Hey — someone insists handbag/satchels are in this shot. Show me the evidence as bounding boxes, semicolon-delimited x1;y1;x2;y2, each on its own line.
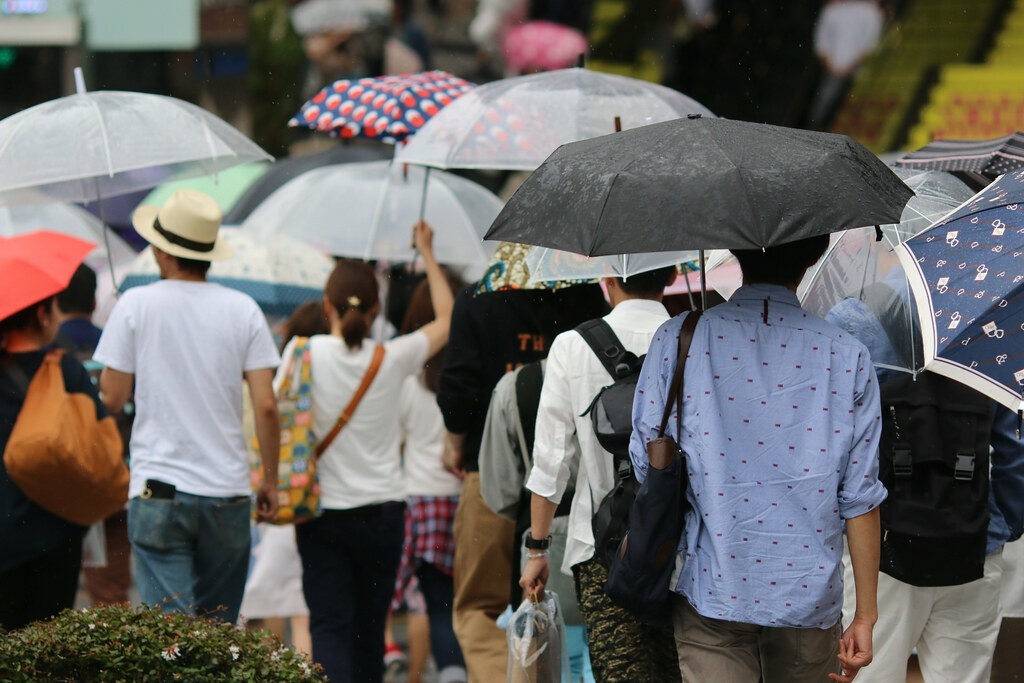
505;588;571;683
3;348;129;525
251;336;322;526
608;309;703;616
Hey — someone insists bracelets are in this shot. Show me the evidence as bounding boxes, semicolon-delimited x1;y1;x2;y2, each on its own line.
526;552;549;558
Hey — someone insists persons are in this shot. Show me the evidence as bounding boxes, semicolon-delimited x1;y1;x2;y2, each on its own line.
518;267;678;683
298;0;880;140
627;235;889;683
275;219;455;683
0;188;1024;683
93;188;283;626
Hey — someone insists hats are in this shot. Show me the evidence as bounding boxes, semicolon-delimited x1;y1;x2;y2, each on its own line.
133;189;233;261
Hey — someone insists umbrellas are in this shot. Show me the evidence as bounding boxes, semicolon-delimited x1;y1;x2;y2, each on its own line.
479;114;917;259
0;53;1024;416
0;67;276;204
243;159;511;267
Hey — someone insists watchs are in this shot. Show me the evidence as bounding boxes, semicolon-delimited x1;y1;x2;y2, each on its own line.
524;530;553;550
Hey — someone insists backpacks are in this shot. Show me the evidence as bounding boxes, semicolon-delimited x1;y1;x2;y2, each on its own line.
575;316;650;566
844;283;994;587
